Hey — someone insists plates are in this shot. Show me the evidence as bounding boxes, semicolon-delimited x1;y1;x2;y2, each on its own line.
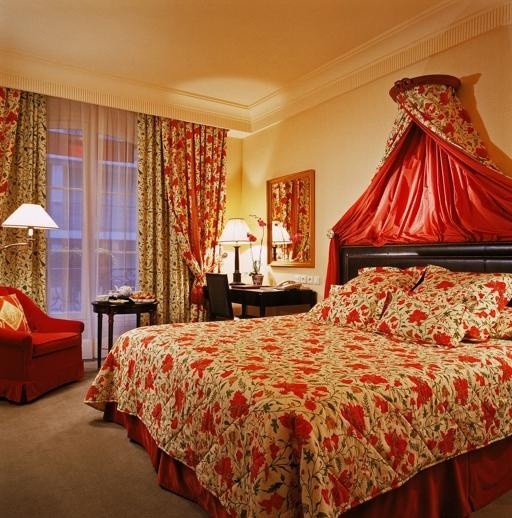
109;299;129;304
129;297;155;304
94;300;108;303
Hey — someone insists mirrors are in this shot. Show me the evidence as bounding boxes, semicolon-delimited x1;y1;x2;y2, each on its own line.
264;169;319;269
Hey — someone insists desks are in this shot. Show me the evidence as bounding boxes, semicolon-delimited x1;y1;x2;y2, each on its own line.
226;282;316;319
92;296;158;375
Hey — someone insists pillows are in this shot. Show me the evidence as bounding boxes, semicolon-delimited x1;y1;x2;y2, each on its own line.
412;265;511;339
311;283;389;332
373;287;489;348
305;264;426;323
488;306;511;341
0;288;33;332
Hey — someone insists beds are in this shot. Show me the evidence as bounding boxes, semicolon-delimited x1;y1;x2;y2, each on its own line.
82;232;512;516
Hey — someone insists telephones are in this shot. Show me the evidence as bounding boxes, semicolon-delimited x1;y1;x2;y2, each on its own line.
276;279;302;292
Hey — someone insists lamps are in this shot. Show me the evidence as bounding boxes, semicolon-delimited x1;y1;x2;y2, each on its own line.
0;201;60;257
271;220;292;260
215;218;254;285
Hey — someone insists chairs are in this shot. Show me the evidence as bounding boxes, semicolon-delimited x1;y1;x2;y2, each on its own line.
204;272;259;322
0;278;87;406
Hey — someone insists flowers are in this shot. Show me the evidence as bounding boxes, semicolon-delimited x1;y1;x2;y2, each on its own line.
246;214;266;270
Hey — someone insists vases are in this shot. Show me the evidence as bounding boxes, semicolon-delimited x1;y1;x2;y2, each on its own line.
252;273;265;288
